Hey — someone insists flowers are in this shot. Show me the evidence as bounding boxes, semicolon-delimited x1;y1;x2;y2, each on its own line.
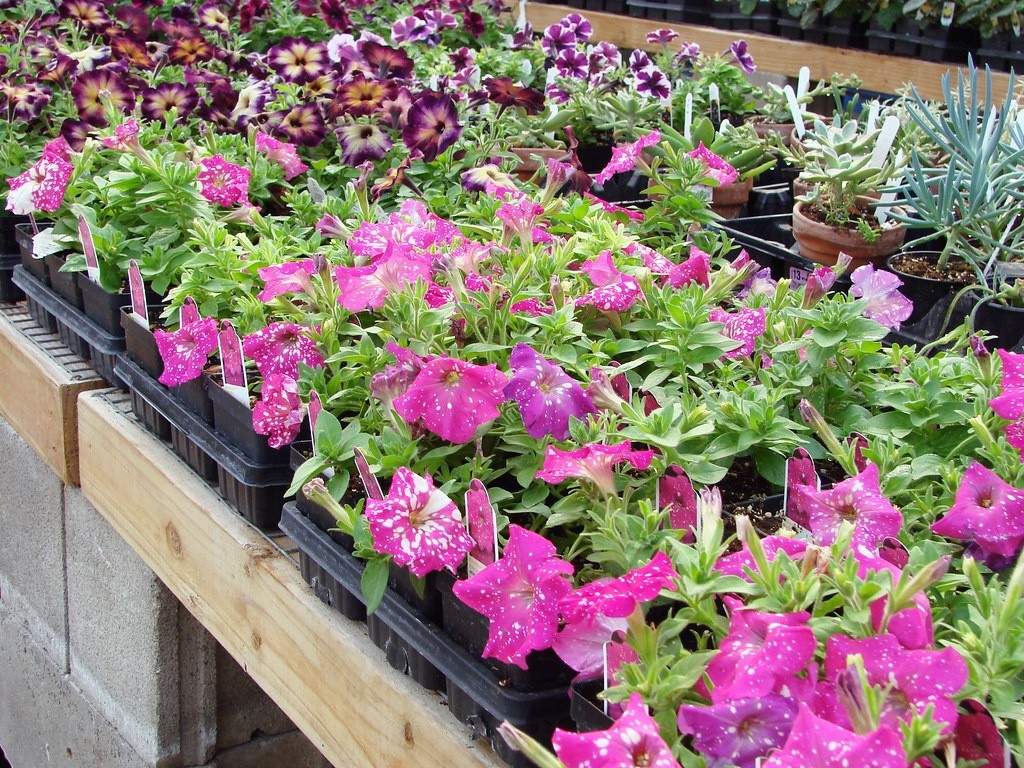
0;0;1024;768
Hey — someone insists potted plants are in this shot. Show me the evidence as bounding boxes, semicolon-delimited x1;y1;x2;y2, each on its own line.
484;51;1024;354
